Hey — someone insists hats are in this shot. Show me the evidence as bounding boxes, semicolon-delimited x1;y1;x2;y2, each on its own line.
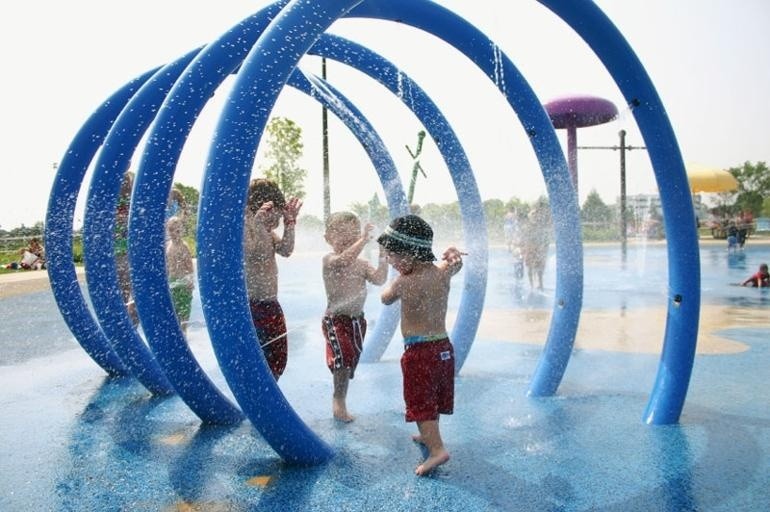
377;213;438;263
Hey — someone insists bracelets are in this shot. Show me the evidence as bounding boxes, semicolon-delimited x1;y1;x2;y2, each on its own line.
283;219;296;225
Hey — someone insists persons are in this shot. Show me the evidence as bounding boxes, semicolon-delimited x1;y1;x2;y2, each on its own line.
114;172;195;346
740;263;770;288
376;214;463;476
241;178;302;384
504;203;551;292
17;238;45;270
708;206;754;250
321;211;389;423
